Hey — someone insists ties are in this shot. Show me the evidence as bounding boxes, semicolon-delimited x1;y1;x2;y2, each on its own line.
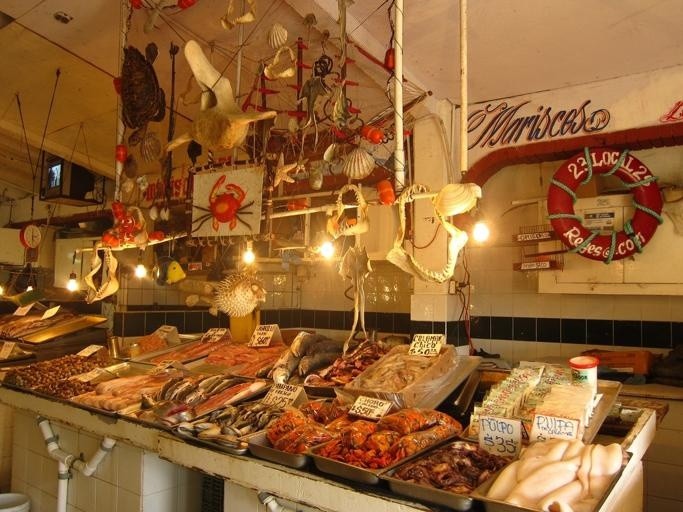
46;158;94;196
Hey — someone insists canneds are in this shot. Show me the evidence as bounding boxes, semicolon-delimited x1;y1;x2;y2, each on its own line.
547;148;663;260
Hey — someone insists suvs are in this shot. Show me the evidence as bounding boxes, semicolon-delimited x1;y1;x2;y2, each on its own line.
0;493;32;512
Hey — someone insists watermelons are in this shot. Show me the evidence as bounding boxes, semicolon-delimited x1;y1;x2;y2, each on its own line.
0;222;44;307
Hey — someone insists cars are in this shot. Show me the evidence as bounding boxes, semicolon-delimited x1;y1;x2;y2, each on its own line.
568;355;599;398
106;335;120;358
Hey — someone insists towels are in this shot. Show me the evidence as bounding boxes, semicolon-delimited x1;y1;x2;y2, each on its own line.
0;492;32;512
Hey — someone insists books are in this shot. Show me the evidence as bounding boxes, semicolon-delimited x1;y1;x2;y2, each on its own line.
342;342;458;413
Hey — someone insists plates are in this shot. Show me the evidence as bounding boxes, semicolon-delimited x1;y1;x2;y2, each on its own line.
536;191;683;296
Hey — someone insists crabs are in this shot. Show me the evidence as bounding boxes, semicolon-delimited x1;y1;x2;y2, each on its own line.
342;148;376;180
267;22;287;49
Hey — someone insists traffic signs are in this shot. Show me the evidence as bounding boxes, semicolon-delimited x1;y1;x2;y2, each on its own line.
449;280;457;295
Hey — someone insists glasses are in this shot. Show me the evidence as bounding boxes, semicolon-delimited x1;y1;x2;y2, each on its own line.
67;250;79;292
243;240;256;263
135;247;146;278
